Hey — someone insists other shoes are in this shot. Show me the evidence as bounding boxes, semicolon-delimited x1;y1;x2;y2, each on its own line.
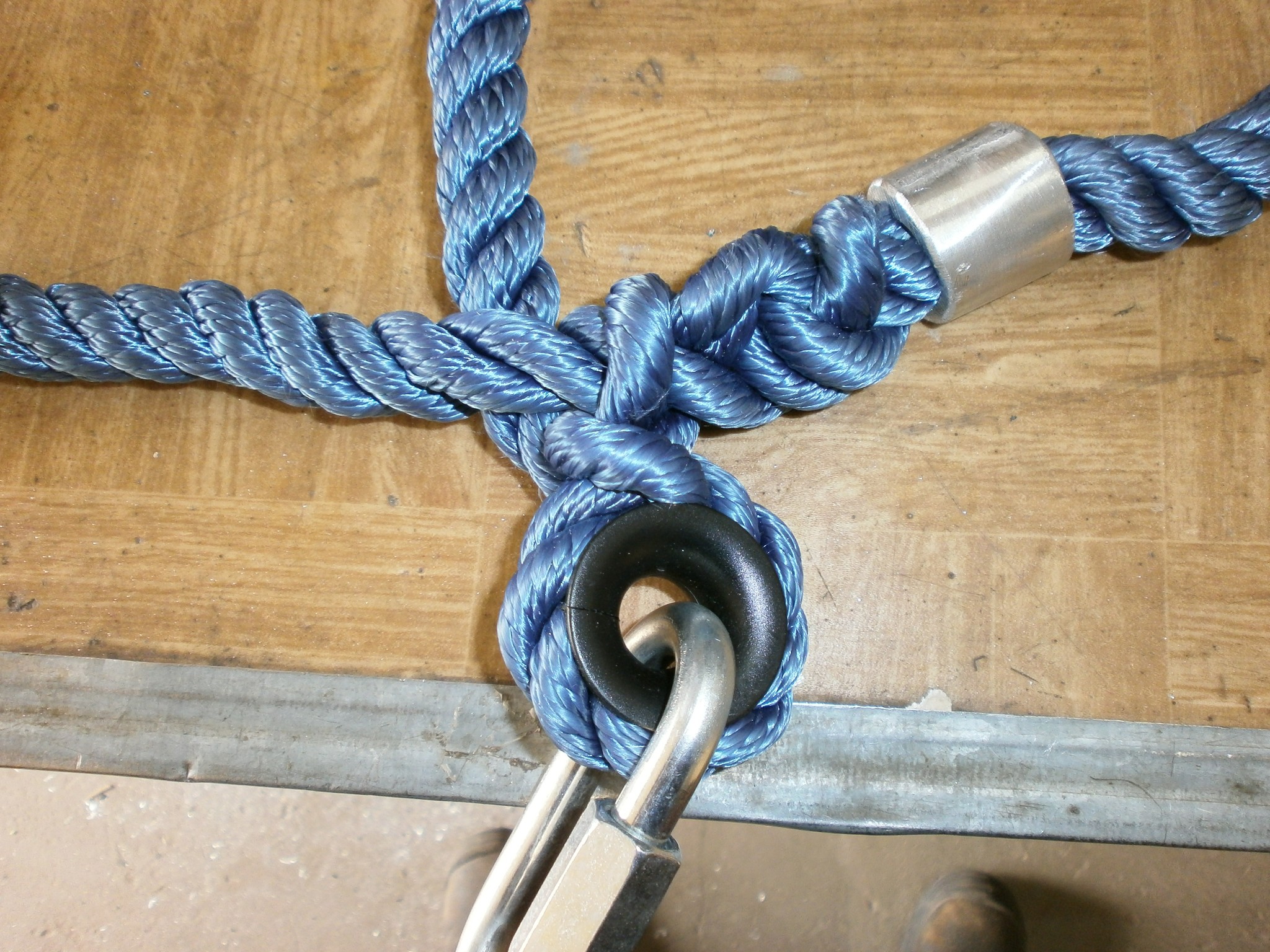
903;868;1025;952
440;827;512;952
